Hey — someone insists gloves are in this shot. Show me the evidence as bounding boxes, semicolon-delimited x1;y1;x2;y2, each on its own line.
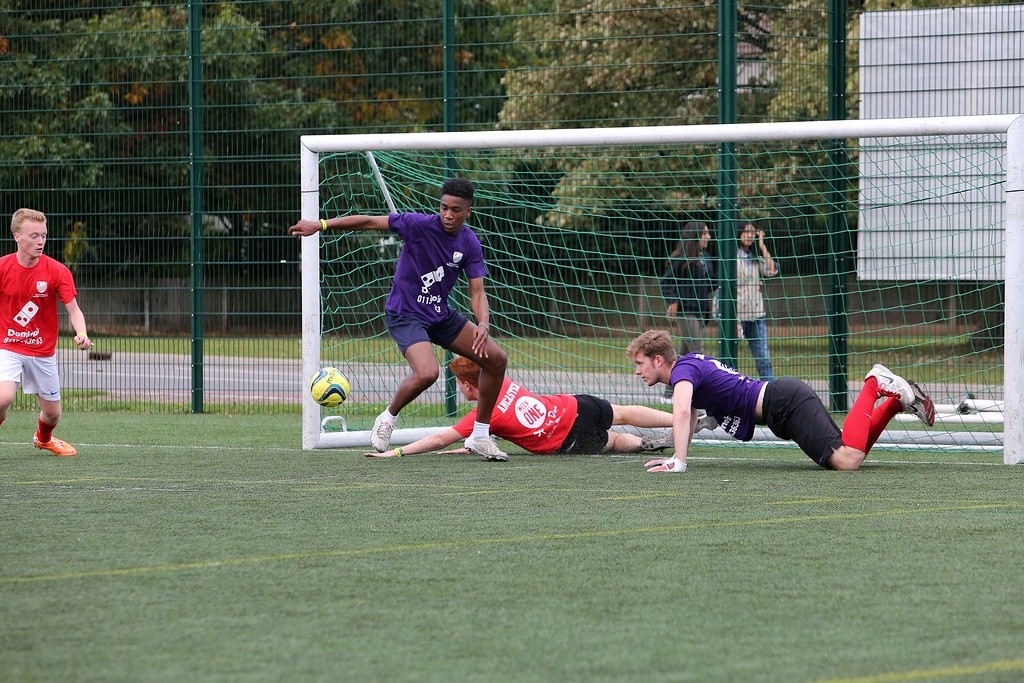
644;453;687;473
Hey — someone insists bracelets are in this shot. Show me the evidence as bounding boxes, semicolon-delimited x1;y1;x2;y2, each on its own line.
320;219;327;231
479;323;489;331
77;333;87;336
394;448;401;457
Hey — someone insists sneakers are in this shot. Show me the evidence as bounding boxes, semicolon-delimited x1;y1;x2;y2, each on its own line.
897;380;935;426
370;412;397;452
864;363;915;405
464;434;509;461
33;432;77;456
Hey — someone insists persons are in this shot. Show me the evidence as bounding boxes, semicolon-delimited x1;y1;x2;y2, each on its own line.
288;180;510;462
715;222;778;381
365;356;718;457
627;330;934;473
661;221;719;403
0;208;91;455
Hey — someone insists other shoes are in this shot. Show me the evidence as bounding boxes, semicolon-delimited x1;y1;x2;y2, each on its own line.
661;397;673;405
640;429;674;451
694;411;717;433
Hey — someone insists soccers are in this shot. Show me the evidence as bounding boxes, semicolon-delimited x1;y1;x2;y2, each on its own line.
310;366;349;407
